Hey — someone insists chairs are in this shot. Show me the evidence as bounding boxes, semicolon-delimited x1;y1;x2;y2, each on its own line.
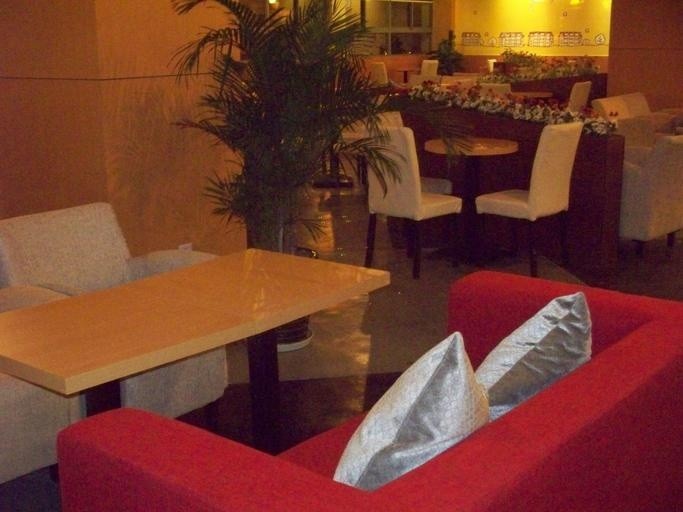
472;119;584;278
364;122;464;281
567;79;683;256
1;203;227;482
0;280;83;486
359;111;451;249
366;58;553;104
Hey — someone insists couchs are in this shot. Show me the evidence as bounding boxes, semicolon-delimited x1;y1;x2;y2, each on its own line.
58;269;683;512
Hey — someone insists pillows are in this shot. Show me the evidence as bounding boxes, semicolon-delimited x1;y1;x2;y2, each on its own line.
333;332;494;493
473;290;592;422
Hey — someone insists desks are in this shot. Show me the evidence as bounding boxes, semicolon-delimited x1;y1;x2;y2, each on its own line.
419;130;519;164
0;250;390;459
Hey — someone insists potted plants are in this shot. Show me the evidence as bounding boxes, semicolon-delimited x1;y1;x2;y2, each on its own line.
158;0;470;352
